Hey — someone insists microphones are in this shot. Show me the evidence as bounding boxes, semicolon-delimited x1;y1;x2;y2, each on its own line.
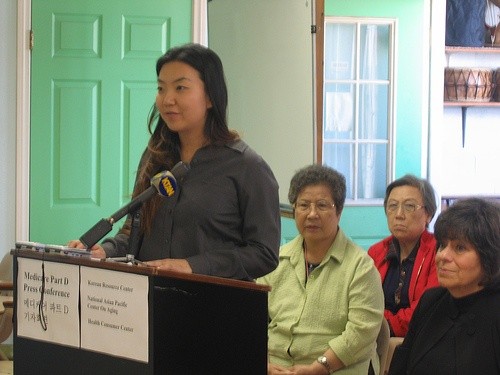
79;159;192;249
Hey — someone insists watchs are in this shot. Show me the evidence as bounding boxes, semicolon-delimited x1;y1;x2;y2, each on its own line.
317;356;333;375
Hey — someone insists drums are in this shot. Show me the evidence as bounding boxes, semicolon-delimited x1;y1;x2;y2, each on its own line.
443;66;496;102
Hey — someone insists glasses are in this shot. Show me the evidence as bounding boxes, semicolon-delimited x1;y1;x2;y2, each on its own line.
294;198;335;212
393;263;409;305
385;199;426;212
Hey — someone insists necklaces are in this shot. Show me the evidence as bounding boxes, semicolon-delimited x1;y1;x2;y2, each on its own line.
304;244;309;276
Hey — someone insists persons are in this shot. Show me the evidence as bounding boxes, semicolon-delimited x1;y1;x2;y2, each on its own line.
256;164;385;375
390;198;500;375
368;175;440;337
68;43;281;280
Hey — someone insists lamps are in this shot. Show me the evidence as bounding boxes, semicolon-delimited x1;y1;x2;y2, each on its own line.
442;67;500;148
